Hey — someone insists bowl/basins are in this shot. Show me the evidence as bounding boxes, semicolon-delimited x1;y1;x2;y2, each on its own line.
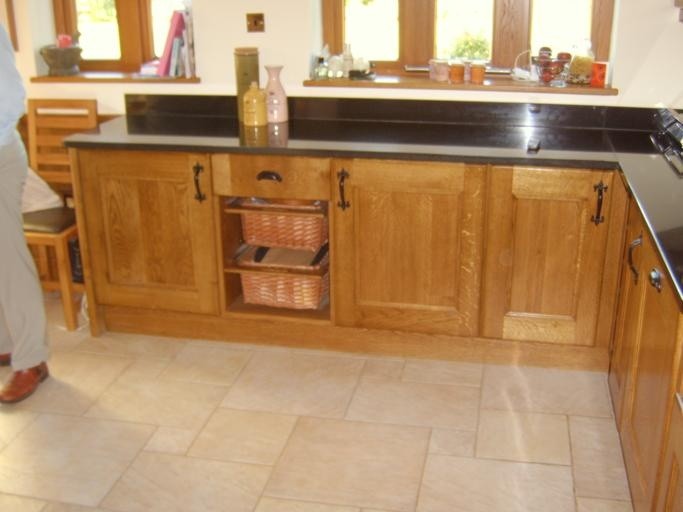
530;56;571;87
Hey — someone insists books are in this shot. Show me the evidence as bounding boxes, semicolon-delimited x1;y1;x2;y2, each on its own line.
156;9;195;80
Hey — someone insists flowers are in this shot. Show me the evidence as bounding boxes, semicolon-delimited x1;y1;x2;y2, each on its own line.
55;30;85;48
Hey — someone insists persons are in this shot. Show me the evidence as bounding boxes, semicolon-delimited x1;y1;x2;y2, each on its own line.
0;25;51;405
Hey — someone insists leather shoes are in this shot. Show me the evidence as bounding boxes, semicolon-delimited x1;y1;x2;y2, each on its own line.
1;352;48;403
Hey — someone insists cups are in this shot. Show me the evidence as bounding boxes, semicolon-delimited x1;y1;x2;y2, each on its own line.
470;64;485;83
450;64;464;83
428;60;450;82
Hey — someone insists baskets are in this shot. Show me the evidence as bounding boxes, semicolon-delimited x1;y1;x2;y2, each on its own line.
236;254;329;310
238;200;329;252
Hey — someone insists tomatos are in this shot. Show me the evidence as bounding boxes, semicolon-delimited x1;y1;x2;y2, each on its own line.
539;47;570;83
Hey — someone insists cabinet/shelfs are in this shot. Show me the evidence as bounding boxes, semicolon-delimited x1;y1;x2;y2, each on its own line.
330;157;486;339
600;197;682;510
72;147;220;318
208;152;336;322
478;165;616;351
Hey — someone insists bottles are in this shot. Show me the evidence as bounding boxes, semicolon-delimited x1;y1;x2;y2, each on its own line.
344;43;354;79
234;47;259;123
312;57;328;80
265;64;288;123
243;81;268;127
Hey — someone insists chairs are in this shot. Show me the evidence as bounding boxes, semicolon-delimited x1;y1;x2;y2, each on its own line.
8;93;103;333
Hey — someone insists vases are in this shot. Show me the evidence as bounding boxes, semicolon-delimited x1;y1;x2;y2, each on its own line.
39;45;81;78
